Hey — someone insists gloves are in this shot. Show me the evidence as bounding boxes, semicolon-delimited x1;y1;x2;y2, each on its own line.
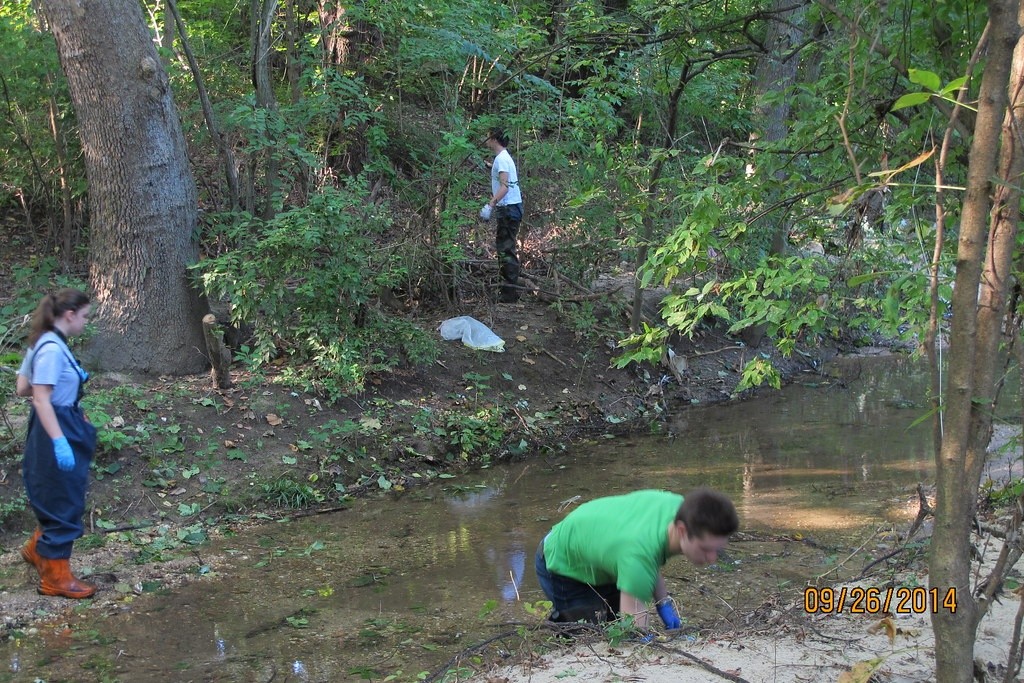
52;436;75;473
655;600;680;630
477;203;494;223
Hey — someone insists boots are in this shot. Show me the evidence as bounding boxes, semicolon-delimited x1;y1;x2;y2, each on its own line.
20;524;43;566
37;555;98;598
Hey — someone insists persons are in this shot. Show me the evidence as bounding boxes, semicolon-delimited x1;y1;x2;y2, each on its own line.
535;489;740;645
479;129;524;303
15;287;96;598
